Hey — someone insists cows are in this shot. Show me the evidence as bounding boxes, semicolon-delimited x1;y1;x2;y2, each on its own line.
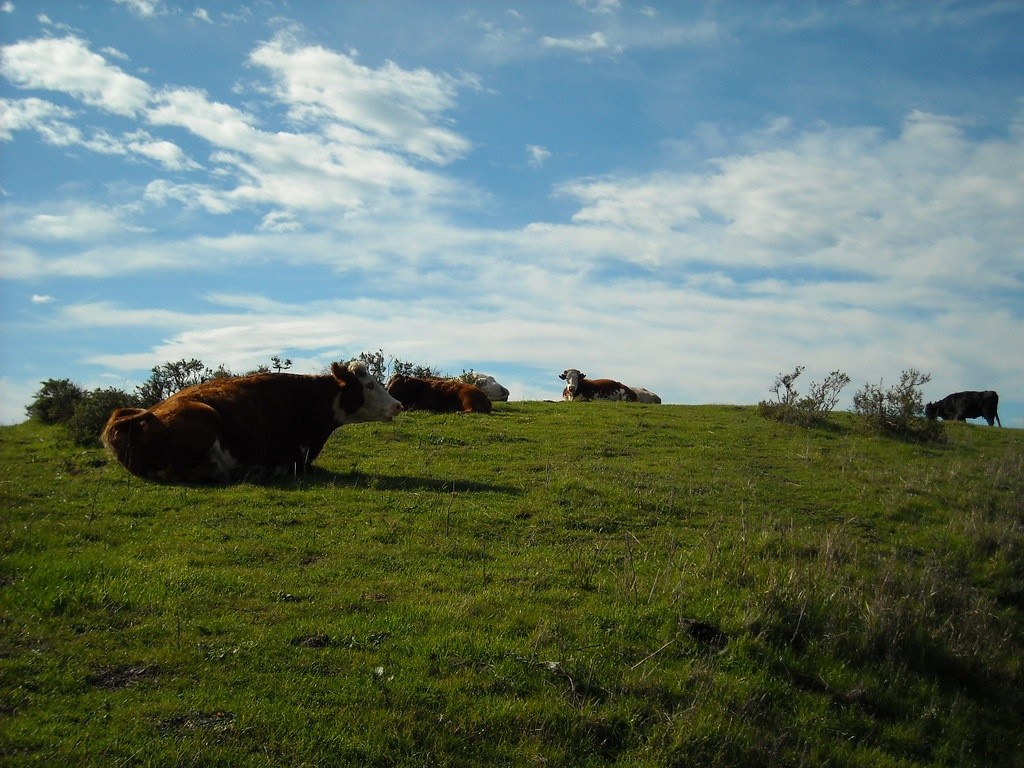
387;373;508;422
558;368;661;403
99;360;405;492
925;390;1001;427
